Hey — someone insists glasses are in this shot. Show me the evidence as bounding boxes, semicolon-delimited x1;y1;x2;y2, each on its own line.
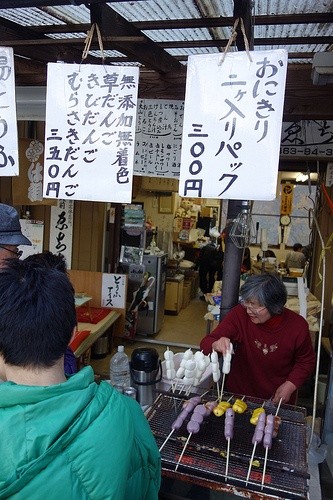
240;298;267;314
0;246;23;259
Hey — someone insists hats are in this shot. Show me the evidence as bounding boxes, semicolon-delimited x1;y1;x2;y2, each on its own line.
0;203;32;246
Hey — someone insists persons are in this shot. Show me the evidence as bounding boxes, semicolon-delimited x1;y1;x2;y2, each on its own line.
285;243;314;274
0;257;164;500
243;248;252;272
200;271;317;403
0;203;79;378
193;241;224;301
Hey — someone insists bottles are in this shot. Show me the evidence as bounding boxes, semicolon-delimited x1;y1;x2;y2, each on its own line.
110;345;131;393
129;348;163;406
124;387;136;400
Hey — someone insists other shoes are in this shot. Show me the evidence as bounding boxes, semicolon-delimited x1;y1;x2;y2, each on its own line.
200;295;206;301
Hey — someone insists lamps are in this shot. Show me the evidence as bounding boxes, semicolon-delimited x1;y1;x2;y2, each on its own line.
310;51;333;86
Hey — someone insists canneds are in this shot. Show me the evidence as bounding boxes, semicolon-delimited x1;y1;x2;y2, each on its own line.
122;387;137;400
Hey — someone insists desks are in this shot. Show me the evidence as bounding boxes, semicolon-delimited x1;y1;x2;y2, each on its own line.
67;305;126;359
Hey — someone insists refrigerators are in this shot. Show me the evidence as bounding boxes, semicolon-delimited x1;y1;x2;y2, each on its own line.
136;253;167;336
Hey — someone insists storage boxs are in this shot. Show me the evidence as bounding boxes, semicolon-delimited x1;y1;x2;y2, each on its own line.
201;207;213;217
164;271;200;316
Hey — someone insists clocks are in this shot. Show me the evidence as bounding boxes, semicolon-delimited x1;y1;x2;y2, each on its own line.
280;214;291;226
